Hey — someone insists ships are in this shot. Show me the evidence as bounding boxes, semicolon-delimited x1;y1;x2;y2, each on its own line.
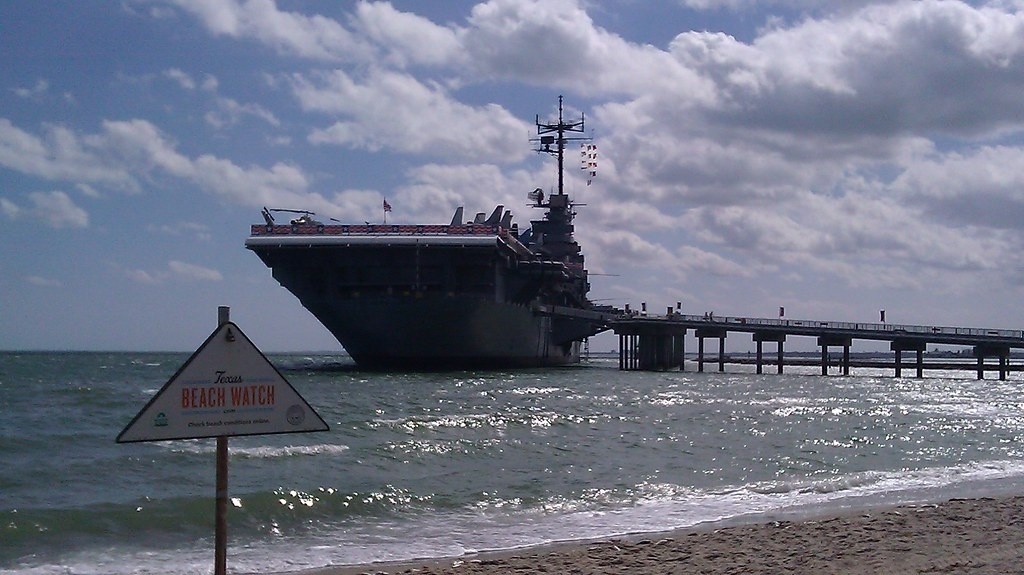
243;94;624;365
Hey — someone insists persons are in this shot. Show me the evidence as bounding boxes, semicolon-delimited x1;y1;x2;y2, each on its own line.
705;311;713;323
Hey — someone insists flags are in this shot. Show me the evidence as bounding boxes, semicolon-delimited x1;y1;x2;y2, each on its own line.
384;200;392;212
581;142;599;186
